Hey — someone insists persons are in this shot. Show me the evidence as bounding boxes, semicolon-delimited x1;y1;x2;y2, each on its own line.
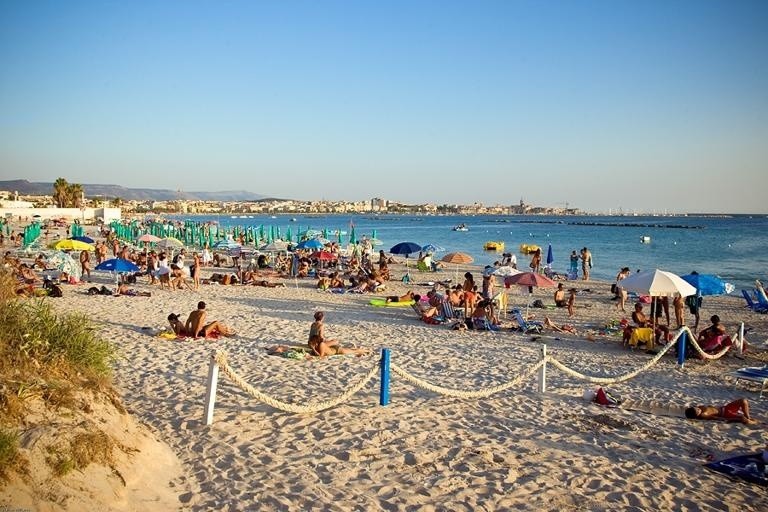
109;230;201;296
555;247;592;319
168;301;236;340
308;311;373;358
615;267;630;313
201;234;390;294
619;297;750;352
684;398;766;425
385;253;517;326
532;248;542;273
2;215;119;297
685;270;703;331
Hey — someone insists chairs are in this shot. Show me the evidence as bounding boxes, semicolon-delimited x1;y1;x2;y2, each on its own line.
728;364;768;396
410;301;456;318
490;292;503;319
741;280;768;315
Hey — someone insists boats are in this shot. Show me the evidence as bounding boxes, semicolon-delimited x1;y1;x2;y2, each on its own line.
322;229;349;235
453;227;470;232
519;243;542;255
483;239;505;251
